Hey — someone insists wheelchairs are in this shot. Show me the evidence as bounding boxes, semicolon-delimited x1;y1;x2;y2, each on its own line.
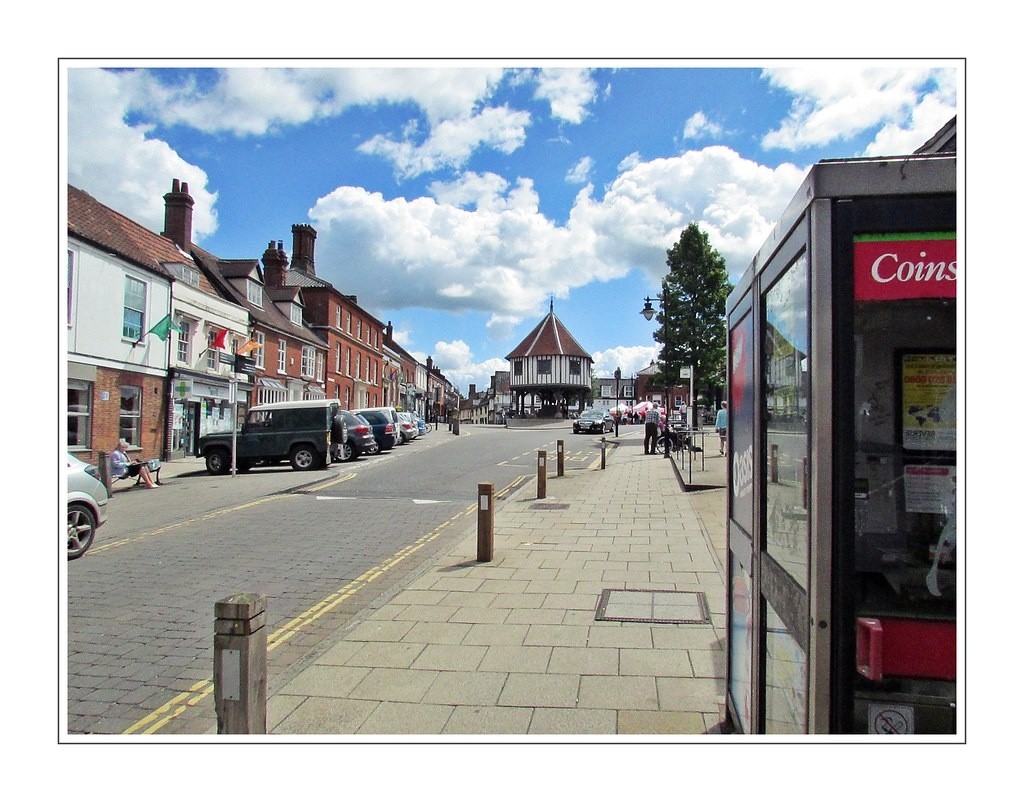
656;422;688;454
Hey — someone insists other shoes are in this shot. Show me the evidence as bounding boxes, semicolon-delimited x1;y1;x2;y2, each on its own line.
650;452;657;455
145;483;160;488
720;449;724;453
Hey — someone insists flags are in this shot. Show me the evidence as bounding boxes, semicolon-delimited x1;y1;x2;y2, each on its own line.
390;369;404;382
149;313;183;341
211;328;226;350
235;338;263;356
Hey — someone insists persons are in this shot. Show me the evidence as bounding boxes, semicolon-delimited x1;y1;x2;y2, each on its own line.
659;415;679;452
109;441;160;489
714;400;727;457
607;406;648;425
644;402;661;455
679;401;688;423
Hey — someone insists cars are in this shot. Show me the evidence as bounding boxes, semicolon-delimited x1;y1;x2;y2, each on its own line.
337;409;376;463
397;412;427;446
354;408;398;456
572;407;616;434
67;451;110;554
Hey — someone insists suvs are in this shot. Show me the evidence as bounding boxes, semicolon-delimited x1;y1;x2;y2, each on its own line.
196;399;347;476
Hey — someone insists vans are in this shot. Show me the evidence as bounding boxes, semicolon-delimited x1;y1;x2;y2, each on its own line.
370;406;400;446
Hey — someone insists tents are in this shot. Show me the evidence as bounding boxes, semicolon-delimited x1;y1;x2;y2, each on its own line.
633;401;664;412
610;403;632;414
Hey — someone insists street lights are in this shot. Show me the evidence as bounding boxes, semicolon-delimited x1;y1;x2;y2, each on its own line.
638;289;671;458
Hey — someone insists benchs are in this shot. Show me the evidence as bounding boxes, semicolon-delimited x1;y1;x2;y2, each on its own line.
111;466;162;485
